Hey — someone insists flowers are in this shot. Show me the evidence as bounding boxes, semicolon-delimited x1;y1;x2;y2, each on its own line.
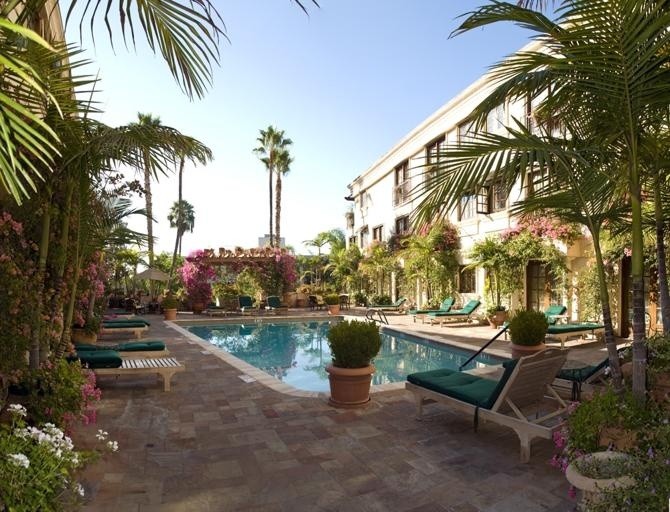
545;380;670;512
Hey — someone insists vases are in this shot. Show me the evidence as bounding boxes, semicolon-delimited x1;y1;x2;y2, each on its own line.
565;451;635;512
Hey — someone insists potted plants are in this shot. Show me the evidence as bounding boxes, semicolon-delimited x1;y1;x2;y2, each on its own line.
324;320;388;409
324;295;341;315
161;293;177;320
509;308;549;366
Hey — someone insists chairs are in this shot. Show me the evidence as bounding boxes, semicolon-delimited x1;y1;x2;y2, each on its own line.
504;305;605;347
406;346;629;466
206;296;288;317
65;308;185;391
366;298;480;327
309;294;350;312
